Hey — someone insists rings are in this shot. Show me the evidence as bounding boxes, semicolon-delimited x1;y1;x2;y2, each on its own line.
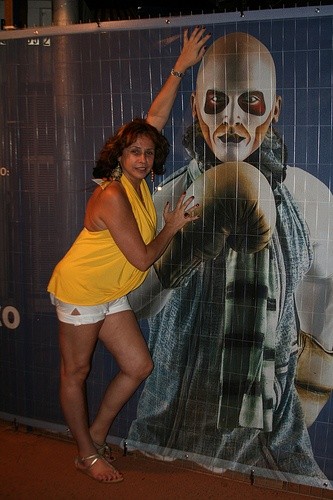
182;212;189;218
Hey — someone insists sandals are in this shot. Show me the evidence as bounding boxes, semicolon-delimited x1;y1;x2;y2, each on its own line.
73;453;124;483
92;440;116;462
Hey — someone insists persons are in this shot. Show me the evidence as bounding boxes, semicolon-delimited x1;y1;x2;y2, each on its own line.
126;31;333;483
45;26;212;484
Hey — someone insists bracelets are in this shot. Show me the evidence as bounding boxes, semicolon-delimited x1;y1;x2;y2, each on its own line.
170;68;183;78
